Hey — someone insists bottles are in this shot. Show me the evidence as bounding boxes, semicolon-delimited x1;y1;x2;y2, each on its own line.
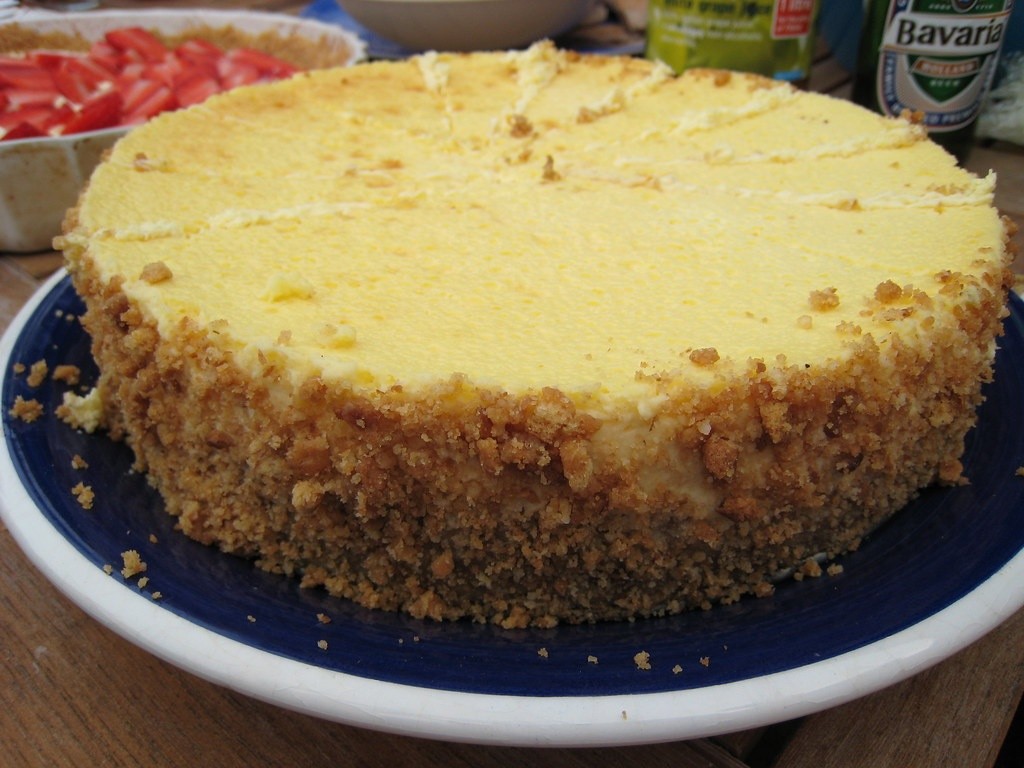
642;0;821;96
853;0;1016;171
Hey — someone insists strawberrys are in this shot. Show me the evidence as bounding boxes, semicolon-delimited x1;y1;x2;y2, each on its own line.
0;23;301;143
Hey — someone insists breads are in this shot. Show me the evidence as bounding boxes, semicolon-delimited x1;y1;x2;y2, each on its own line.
54;36;1016;635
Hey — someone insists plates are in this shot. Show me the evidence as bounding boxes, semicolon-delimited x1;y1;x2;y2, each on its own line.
0;216;1023;755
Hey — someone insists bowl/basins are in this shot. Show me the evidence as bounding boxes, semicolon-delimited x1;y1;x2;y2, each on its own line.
0;9;364;258
330;0;595;51
